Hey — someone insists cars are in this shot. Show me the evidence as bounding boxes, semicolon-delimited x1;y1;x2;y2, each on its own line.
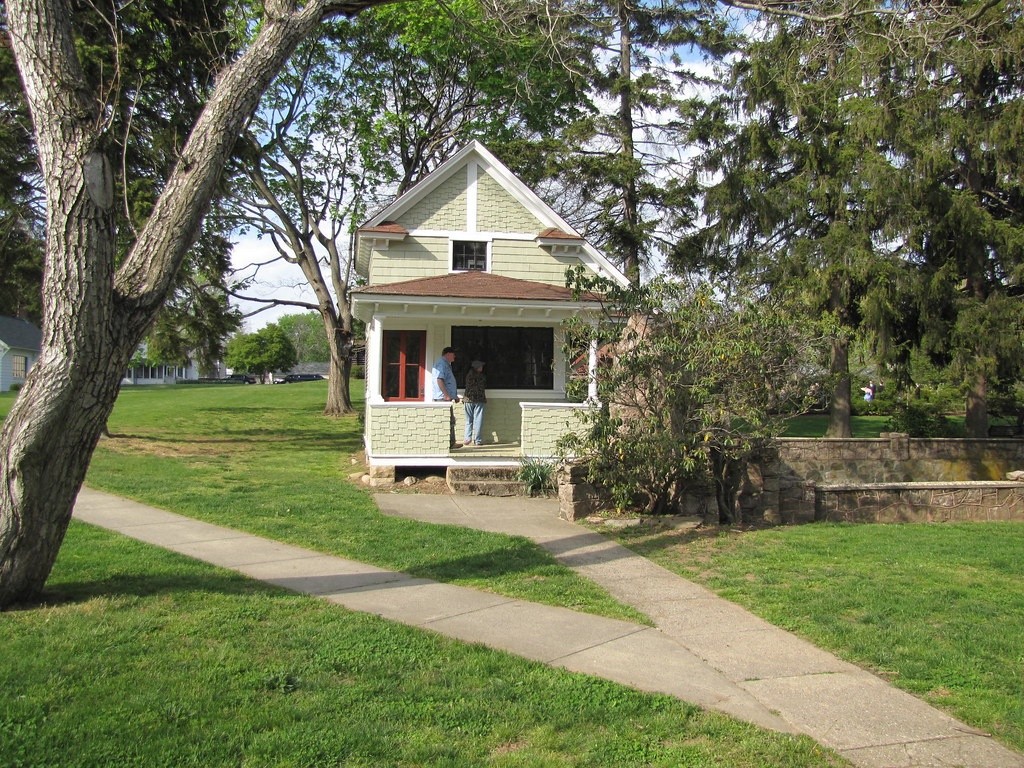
272;378;286;385
230;374;256;384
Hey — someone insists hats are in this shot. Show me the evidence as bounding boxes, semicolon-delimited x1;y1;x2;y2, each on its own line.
442;347;456;356
471;360;485;369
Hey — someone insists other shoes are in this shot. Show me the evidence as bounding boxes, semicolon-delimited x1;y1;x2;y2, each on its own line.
464;439;471;445
475;443;483;445
451;443;463;449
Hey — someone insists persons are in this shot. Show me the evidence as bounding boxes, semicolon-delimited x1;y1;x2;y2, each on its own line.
915;383;921;400
861;379;884;401
431;347;464;449
458;334;494;389
463;360;488;446
